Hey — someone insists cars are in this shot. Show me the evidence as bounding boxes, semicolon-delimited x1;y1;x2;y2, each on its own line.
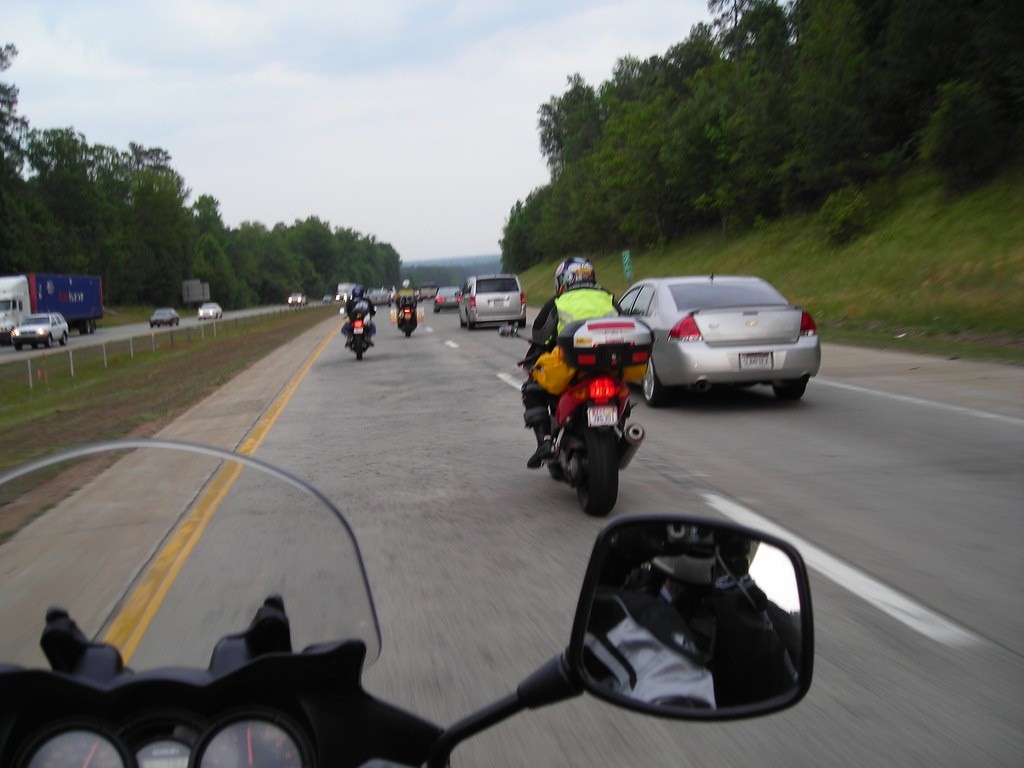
616;274;821;408
414;283;464;313
322;281;357;303
369;288;392;307
148;307;180;328
198;302;223;319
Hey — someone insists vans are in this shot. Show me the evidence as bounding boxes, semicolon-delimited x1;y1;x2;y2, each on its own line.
455;273;526;332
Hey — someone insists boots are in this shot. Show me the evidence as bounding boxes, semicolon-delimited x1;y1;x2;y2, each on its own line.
527;419;555;469
366;334;374;346
345;332;353;346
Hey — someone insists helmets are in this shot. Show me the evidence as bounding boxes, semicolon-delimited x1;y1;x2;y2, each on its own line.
402;279;411;289
554;257;595;295
352;286;364;297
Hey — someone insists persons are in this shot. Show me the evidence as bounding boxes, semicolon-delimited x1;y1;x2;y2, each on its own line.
521;255;628;470
393;279;417;328
341;286;376;347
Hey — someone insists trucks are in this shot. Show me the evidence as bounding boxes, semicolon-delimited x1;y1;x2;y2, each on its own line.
0;272;104;345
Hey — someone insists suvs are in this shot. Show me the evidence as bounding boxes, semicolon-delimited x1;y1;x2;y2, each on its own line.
288;291;312;308
11;313;69;350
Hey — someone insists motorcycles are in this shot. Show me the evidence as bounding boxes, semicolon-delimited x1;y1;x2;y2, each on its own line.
396;296;418;338
347;306;377;360
528;315;656;517
